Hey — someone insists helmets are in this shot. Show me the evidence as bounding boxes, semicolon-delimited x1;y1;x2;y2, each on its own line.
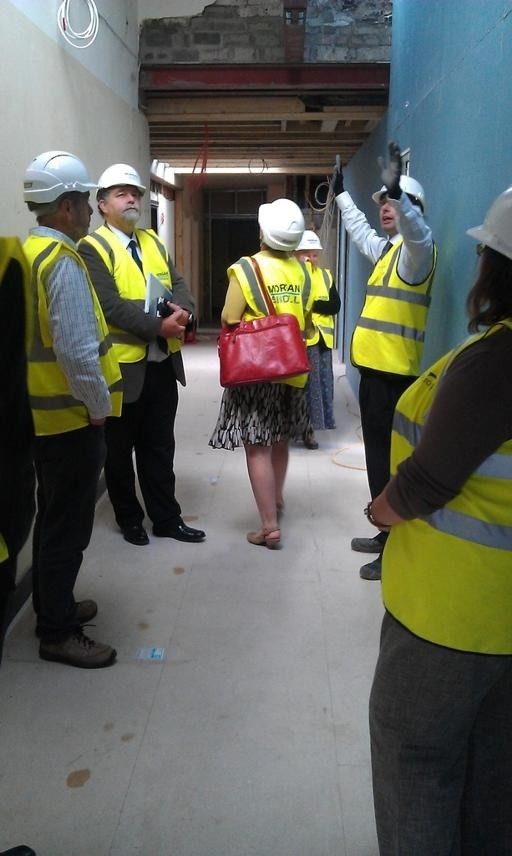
466;187;512;259
258;199;305;252
21;150;104;214
371;175;425;212
95;163;147;203
296;230;323;251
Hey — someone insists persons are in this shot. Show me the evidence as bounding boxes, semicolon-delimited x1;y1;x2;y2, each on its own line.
76;164;208;544
372;185;509;849
212;199;306;549
285;229;340;449
327;142;434;580
19;151;126;670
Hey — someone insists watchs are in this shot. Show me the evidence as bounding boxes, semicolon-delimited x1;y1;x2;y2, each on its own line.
364;501;392;527
188;309;195;328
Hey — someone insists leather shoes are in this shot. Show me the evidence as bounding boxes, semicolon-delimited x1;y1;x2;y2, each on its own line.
123;525;149;545
303;431;318;450
153;521;207;542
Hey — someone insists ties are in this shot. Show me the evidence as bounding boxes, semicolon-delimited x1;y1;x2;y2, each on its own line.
129;241;143;273
380;241;393;256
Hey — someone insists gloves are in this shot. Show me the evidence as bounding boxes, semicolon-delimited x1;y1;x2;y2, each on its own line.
378;141;403;198
326;154;344;196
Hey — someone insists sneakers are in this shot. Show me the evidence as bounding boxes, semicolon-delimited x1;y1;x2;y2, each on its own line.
38;633;117;670
74;599;98;622
352;535;383;552
360;555;384;580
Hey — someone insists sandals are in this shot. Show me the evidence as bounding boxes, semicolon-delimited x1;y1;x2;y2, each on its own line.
276;503;284;520
245;527;283;548
